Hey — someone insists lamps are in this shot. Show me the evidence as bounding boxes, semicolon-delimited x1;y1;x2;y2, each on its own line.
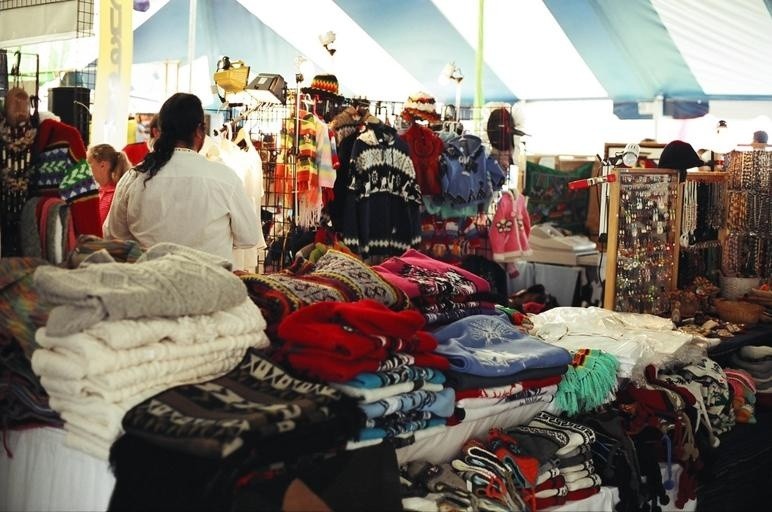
242;73;287;105
438;59;464;120
622;143;640;168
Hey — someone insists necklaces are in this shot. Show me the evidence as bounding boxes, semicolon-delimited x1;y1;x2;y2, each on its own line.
679;150;772;280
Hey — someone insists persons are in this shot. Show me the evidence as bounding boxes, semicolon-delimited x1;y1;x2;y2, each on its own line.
121;112;161;167
86;143;129;226
751;131;768;144
106;92;260;271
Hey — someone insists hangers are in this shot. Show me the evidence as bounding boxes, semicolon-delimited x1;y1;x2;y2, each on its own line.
225;113;253;148
305;93;313;113
434;121;475;146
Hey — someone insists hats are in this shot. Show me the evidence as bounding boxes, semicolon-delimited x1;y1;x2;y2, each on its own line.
401;410;603;512
563;362;757;512
401;93;440;123
301;75;344;103
658;141;704;169
